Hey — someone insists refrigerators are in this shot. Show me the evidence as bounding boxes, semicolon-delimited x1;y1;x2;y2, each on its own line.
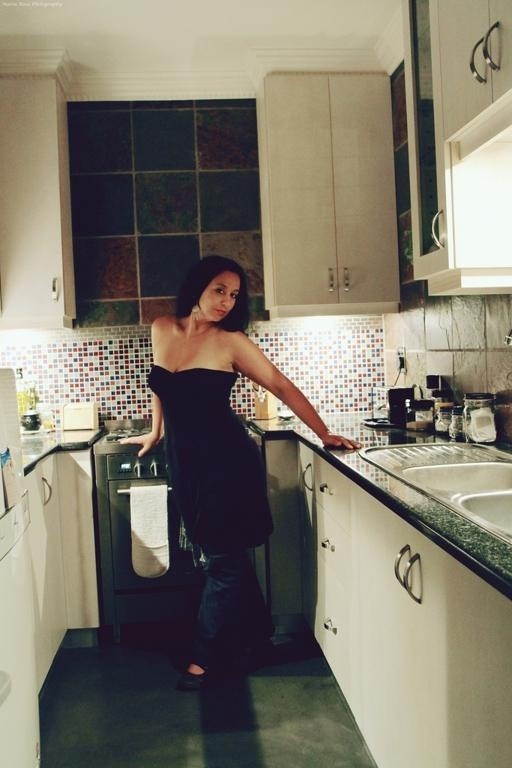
0;364;42;767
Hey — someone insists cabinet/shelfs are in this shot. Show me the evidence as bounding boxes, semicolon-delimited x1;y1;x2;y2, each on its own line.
402;1;512;296
251;67;402;317
0;49;79;336
253;429;349;705
436;1;512;162
25;445;100;701
350;486;511;767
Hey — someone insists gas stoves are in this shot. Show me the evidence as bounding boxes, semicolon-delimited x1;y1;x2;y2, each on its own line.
93;426;264;480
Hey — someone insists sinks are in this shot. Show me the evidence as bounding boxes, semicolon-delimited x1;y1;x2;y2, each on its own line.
398;461;512;496
451;491;512;546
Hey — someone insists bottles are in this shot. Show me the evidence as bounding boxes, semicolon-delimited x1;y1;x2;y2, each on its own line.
426;374;439;402
462;391;512;443
431;388;442;422
406;399;433;431
449;406;465;439
436;404;450;432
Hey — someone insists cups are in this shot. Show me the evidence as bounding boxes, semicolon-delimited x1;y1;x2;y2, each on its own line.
20;413;40;432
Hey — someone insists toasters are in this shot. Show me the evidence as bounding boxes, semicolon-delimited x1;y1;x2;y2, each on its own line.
364;384;415;429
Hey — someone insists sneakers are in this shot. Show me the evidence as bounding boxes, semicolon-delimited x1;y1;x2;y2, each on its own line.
177;663;212;690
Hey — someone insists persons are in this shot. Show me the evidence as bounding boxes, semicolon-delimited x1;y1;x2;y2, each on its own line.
117;254;366;694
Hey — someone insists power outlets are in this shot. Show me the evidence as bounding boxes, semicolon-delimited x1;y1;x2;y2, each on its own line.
396;345;408;374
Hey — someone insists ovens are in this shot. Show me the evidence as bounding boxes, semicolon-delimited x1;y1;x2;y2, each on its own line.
93;481;267;647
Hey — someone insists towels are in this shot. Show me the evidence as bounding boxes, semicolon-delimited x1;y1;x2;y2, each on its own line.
128;485;170;579
